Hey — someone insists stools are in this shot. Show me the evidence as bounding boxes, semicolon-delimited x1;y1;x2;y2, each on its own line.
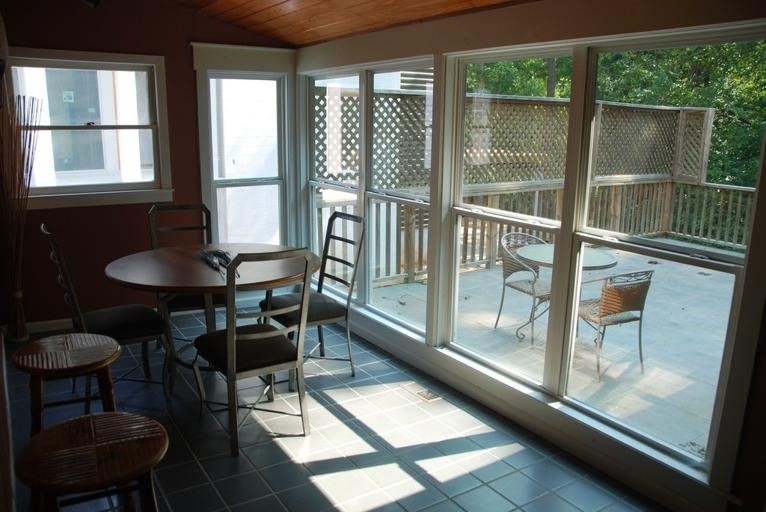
9;333;173;510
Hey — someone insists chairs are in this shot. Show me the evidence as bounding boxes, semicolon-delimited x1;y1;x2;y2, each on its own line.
258;210;370;392
193;248;314;459
148;203;227;349
492;232;655;380
42;223;172;415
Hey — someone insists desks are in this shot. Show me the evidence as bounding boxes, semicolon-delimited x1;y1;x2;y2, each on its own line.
104;243;321;422
